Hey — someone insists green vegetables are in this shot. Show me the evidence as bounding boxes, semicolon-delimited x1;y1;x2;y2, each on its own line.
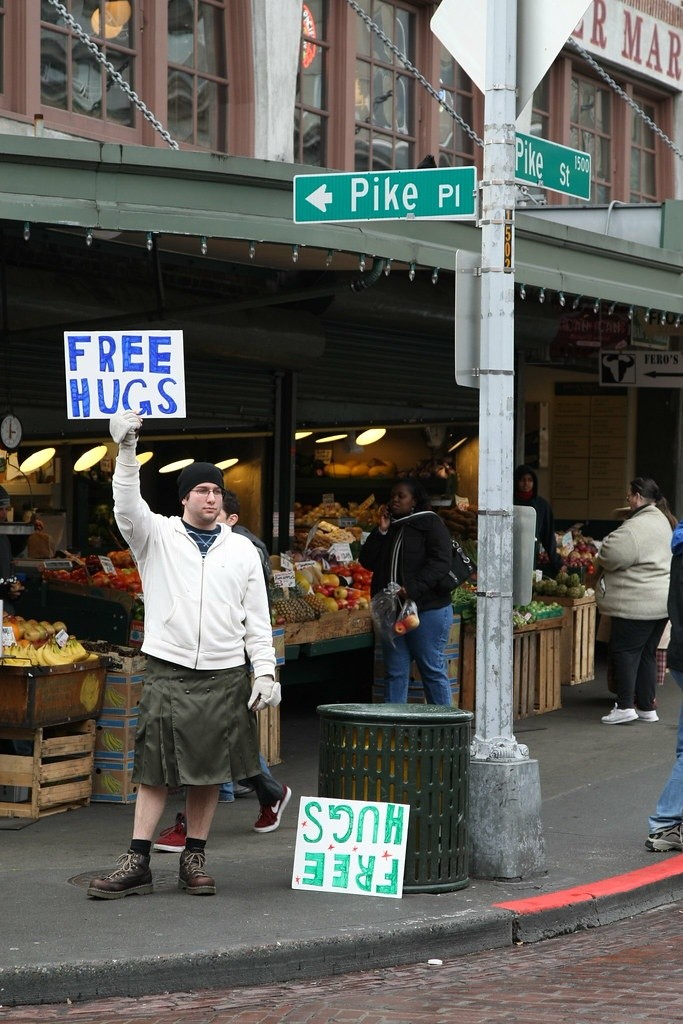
514;600;561;629
450;532;478;604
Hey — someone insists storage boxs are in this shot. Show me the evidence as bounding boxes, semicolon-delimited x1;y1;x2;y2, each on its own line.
318;516;356;527
250;627;285;673
283;607;373;646
75;639;146;804
0;718;97;820
370;613;461;708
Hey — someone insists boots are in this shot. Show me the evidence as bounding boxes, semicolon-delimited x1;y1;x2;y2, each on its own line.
87;849;153;899
178;847;217;894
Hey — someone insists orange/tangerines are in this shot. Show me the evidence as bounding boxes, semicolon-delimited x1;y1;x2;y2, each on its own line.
135;605;144;621
4;623;20;641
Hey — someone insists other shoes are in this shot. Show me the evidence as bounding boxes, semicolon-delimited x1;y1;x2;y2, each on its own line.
233;783;255;795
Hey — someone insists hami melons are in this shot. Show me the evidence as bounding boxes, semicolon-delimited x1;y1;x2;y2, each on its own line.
329;458;393;476
269;553;322;574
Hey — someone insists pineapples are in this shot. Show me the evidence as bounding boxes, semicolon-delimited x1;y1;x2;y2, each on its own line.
269;581;329;623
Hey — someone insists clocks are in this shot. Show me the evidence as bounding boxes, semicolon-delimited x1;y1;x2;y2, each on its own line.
0;413;23;451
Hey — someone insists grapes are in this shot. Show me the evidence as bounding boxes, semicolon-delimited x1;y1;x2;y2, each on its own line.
81;641;148;659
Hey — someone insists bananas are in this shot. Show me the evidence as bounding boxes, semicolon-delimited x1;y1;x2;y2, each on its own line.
2;635;98;666
102;686;127;794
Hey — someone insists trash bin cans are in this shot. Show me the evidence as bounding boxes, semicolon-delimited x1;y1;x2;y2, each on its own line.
317;704;475;894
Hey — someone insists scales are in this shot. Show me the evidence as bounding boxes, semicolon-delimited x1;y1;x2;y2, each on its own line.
15;557;73;571
0;413;36;534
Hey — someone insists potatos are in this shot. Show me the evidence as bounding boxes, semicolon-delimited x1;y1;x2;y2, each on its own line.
437;509;477;531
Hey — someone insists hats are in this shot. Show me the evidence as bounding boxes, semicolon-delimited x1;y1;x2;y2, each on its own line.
177;462;226;502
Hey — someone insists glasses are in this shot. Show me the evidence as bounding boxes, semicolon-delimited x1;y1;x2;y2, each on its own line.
190;488;225;496
626;493;635;501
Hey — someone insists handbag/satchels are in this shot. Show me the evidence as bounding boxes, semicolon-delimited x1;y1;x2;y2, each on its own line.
424;529;474;598
368;582;419;648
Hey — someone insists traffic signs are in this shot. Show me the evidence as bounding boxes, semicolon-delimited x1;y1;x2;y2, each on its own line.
294;165;480;224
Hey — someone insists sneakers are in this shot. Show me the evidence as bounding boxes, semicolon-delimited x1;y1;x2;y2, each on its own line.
253;784;292;833
645;823;683;852
153;812;187;852
635;707;659;722
601;702;639;724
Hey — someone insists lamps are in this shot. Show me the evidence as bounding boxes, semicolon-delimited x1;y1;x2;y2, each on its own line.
314;428;387;446
71;443;108;472
156;445;194;473
206;440;239;470
18;446;56;472
446;431;469;453
116;442;153;467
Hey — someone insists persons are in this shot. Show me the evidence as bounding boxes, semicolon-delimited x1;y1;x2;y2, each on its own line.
88;411;274;895
154;490;292;853
359;479;454;705
513;465;560;567
594;478;678;725
0;485;43;614
645;517;683;850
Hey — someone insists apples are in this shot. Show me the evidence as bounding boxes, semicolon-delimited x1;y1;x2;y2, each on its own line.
294;502;388;525
44;549;143;592
312;562;373;610
539;543;597;574
393;614;419;635
3;608;67;641
